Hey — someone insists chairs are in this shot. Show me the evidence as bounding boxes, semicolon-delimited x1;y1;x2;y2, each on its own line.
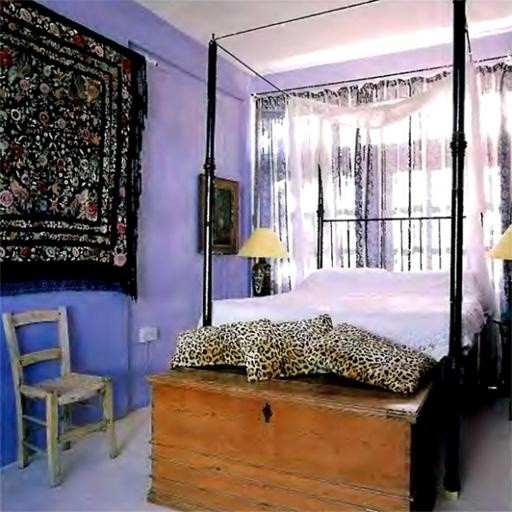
2;305;117;489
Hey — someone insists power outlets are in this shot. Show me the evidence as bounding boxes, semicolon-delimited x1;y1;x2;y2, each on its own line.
138;325;159;343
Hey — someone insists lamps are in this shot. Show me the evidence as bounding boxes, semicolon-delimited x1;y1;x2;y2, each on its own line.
487;222;512;319
237;226;291;297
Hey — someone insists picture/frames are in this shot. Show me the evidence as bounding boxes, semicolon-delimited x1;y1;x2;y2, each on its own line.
199;173;240;254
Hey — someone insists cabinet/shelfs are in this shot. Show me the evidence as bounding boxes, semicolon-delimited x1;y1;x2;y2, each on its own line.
142;363;444;512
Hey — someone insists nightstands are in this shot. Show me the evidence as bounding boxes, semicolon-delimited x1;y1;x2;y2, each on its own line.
484;313;512;422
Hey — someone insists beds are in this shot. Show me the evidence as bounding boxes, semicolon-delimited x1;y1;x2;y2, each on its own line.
201;0;495;501
